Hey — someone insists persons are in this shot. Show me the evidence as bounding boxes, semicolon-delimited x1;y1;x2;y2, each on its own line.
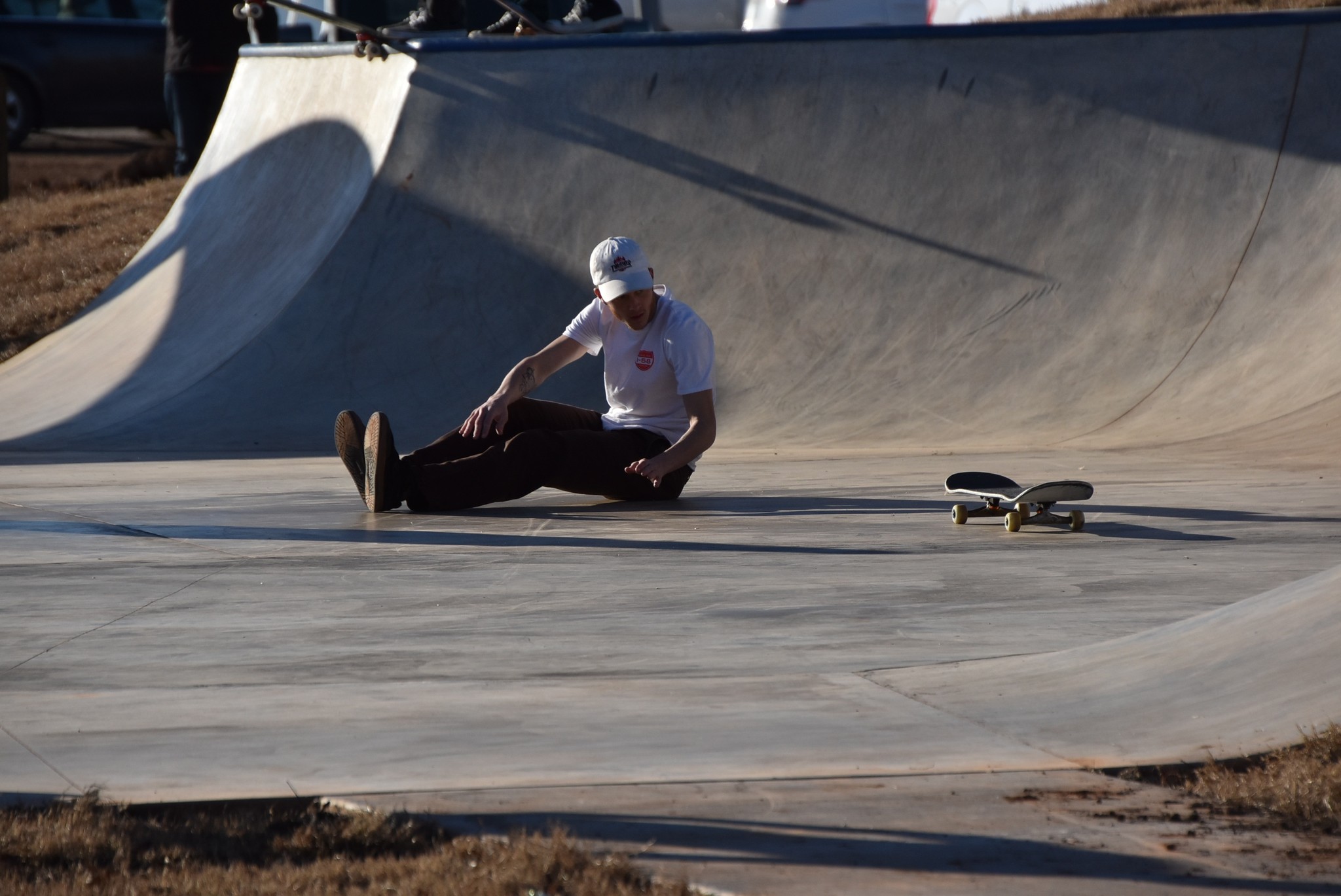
334;236;717;515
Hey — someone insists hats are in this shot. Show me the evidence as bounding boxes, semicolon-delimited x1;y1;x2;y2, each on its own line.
587;236;655;304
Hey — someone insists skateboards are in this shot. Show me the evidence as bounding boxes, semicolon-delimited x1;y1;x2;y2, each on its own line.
945;471;1094;532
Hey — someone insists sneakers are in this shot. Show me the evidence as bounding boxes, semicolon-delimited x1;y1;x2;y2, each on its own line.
334;409;367;507
465;9;519;38
362;411;404;514
377;6;469;41
544;0;623;34
